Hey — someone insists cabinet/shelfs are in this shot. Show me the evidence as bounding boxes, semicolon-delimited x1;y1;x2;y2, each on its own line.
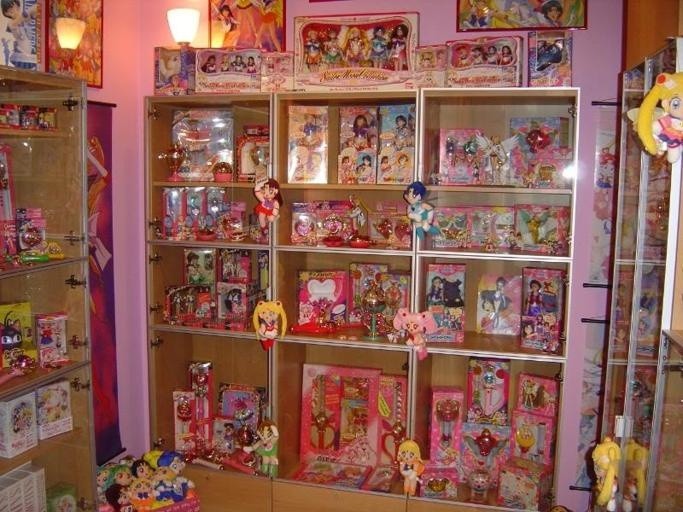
271;90;420;500
142;93;274;481
0;64;101;512
409;87;582;512
570;34;683;512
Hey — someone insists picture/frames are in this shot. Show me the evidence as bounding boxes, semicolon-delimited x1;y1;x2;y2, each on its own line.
207;0;287;53
44;0;104;89
454;0;589;33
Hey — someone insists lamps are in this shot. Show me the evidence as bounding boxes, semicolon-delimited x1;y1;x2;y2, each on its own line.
164;6;202;45
51;11;89;62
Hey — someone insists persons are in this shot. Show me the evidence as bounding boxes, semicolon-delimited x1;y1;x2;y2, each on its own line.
592;436;620;512
627;71;683;163
393;308;437;361
96;449;196;512
243;419;280;479
622;440;647;511
403;181;441;241
254;164;283;236
253;300;287;351
396;440;425;495
